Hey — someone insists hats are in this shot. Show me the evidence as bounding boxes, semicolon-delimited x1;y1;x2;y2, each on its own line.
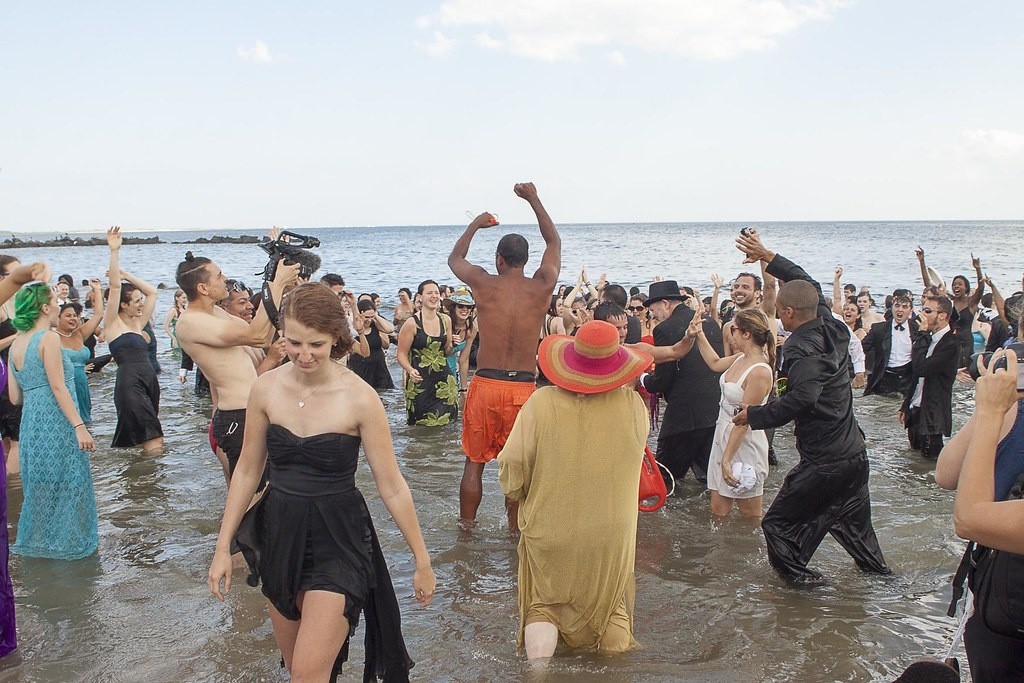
538;320;654;393
443;287;476;312
643;280;691;308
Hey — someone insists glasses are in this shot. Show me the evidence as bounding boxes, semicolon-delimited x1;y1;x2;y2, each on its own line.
921;307;942;314
572;306;588;314
229;281;244;295
628;305;647;311
455;304;472;309
335;291;345;298
730;325;743;336
910;297;914;302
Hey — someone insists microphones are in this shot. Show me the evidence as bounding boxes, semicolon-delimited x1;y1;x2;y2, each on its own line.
277;244;321;271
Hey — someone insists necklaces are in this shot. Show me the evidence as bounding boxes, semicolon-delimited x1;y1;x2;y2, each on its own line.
288;366;337;408
56;331;71;338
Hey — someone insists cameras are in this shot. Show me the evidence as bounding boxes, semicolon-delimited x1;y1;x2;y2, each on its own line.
968;340;1024;392
734;407;743;416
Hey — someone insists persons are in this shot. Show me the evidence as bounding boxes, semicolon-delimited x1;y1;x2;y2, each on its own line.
637;280;727;485
498;319;651;667
0;354;17;659
0;226;1024;513
205;283;436;683
935;348;1024;682
7;282;98;559
696;309;774;517
448;182;562;532
174;251;300;506
733;229;889;582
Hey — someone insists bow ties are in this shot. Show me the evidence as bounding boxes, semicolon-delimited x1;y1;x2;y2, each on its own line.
894;323;905;330
